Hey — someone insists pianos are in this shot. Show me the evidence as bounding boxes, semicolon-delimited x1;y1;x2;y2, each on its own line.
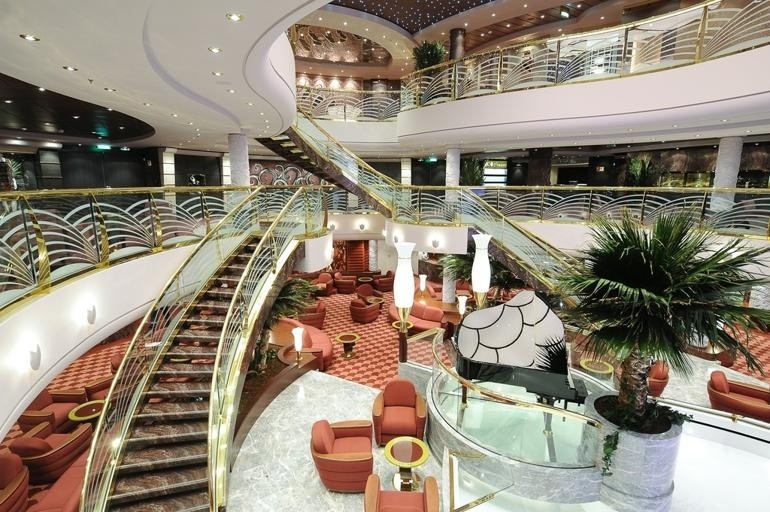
443;286;588;430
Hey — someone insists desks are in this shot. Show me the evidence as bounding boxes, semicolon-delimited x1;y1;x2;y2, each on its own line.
335;333;361;359
391;320;414;342
580;359;614;381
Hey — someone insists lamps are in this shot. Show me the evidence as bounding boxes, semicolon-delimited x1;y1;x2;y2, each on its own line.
471;232;492;311
393;241;415;364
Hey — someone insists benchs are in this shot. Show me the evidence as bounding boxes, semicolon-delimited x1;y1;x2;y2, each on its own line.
389;300;444;341
295;320;333;371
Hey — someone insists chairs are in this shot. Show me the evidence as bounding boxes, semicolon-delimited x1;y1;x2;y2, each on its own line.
299;271;394;329
707;370;770;423
310;379;439;512
645;359;671;398
0;350;152;511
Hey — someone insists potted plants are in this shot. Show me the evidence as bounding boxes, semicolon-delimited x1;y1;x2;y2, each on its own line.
541;188;770;508
